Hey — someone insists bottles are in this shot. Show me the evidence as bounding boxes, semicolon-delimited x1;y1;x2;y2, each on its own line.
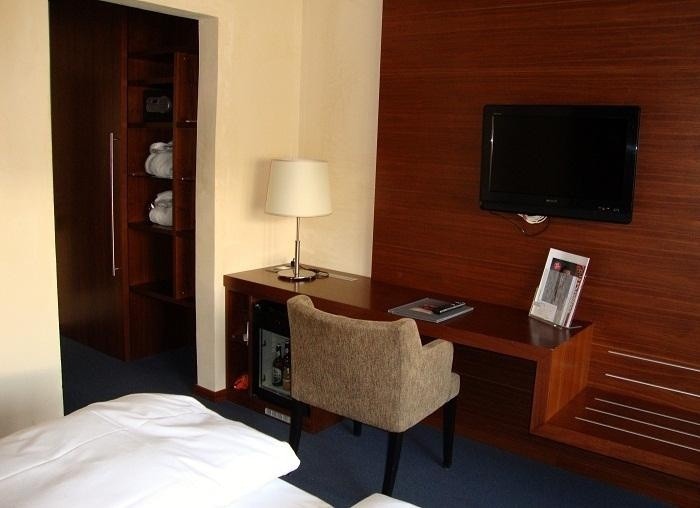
272;337;292;393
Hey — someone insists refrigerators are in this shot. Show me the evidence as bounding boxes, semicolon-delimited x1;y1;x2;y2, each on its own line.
249;304;309;417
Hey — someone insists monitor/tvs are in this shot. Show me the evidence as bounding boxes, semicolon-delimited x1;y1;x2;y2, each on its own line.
480;104;641;224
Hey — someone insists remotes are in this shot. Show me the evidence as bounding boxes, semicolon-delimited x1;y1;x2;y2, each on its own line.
432;300;466;314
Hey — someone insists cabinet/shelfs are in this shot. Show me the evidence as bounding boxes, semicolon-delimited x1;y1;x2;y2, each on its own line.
47;0;201;364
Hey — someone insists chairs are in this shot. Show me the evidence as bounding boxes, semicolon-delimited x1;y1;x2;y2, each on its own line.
284;293;464;494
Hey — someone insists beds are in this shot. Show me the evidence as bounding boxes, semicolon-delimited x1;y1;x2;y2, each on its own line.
2;391;421;508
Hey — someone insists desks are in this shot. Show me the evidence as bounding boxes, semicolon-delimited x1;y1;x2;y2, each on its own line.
220;258;596;432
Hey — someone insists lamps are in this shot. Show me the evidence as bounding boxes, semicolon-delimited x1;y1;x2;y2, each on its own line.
262;157;333;281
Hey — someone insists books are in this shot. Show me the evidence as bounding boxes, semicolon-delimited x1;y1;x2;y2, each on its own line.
389;296;473;323
